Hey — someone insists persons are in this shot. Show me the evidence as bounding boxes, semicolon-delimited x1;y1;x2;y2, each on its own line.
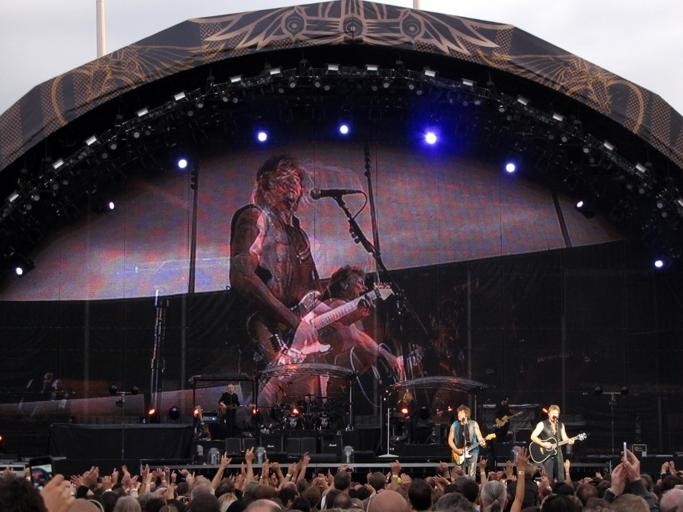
0;441;682;512
529;402;577;484
218;383;242;437
19;371;75;426
447;402;487;484
491;394;519;450
229;152;426;414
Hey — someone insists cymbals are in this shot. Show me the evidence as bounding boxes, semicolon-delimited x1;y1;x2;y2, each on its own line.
390;377;496;391
259;364;359;378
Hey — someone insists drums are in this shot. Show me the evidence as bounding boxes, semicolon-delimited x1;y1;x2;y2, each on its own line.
409;386;472;435
279;370;349;415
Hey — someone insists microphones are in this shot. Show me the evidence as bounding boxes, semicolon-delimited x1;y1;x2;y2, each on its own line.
461;418;466;426
309;187;362;201
552;415;558;422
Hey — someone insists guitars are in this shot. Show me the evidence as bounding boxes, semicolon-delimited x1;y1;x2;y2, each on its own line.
246;284;394;376
496;411;523;426
529;433;588;464
452;434;496;465
